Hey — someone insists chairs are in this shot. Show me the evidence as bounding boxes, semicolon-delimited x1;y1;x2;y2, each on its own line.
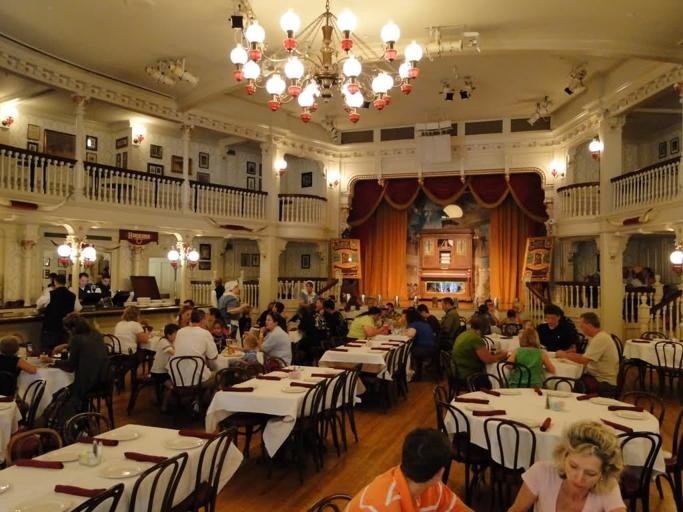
640;331;666;339
656;410;683;512
466;371;502;393
610;333;646;390
543;376;587;391
500;323;523;336
71;483;126;512
215;367;260;459
655;341;683;399
193;426;238;512
169;356;210;425
496;358;532;389
433;384;450;431
300;362;363;472
483;417;537;512
377;338;414;414
439;350;480;400
129;452;189;512
19;379;47;428
574;332;585;353
436;400;491;507
622;391;666;427
61;408;116;444
103;334;122;354
4;432;67;458
305;492;351;510
482;336;494;349
127;346;165;416
615;430;664;512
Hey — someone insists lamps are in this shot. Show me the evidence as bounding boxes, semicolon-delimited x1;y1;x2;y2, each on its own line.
550;160;562;179
57;244;72;268
669;251;683;275
133;128;146;144
230;0;424;123
588;141;600;161
3;106;18;127
188;246;200;272
166;246;180;268
278;160;288;177
81;244;97;270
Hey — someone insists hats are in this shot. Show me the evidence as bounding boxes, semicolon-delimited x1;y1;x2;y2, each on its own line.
225;280;237;291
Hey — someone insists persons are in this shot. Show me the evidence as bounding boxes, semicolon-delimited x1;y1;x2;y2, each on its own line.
406;307;435;358
210;319;227;354
44;311;112;412
501;309;523;336
439;297;461;351
214;277;225;299
297;307;315;350
149;323;180;380
96;275;110;298
323;300;349;338
239;306;252;337
505;327;554;387
418;304;440;333
344;427;474;511
178;305;193;328
346;306;389;341
0;335;37;398
165;310;218;387
218;280;251;318
382;303;401;320
555;312;621;397
269;303;288;334
345;296;361;311
314;298;326;330
207;308;226;325
536;304;579;351
485;299;498;323
299;282;318;308
449;317;492;391
44;275;76;336
471;305;496;331
109;306;149;364
258;314;293;368
36;273;58;309
184;299;194;307
508;420;628;512
79;273;89;300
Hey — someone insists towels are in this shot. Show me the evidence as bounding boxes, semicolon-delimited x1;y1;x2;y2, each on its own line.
576;392;599;402
631;339;650;344
256;374;281;380
344;343;361;347
328;348;348;352
351;339;367;343
80;435;120;446
221;387;254;393
54;484;106;498
454;397;489;404
289;381;315;388
471;409;506;416
534;385;542;395
311;373;338;378
389;339;405;343
124;451;168;464
179;429;218;440
601;418;633;433
539;417;551;432
381;342;400;346
479;387;500;396
16;457;63;470
0;397;14;403
607;404;644;413
272;368;295;372
371;346;390;351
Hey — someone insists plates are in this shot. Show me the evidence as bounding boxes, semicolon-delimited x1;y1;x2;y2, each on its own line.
101;429;140;441
616;409;647;422
499;388;522;396
43;448;80;462
0;480;10;493
591;396;618;406
465;403;494;411
547;390;572;398
506;416;539;429
97;460;148;479
13;495;75;512
282;386;304;394
164;435;204;449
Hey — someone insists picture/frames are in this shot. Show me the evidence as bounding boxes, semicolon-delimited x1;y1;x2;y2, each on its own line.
241;253;252;267
115;136;128;149
259;163;262;177
149;144;163;159
246;161;256;175
147;162;165;177
171;154;193;176
27;141;40;152
300;171;313;188
657;140;668;158
27;123;41;141
196;172;210;184
259;178;262;191
199;261;213;270
86;152;97;164
251;254;260;267
301;254;311;269
669;137;680;155
247;177;256;190
199;243;212;260
199;152;209;169
122;151;127;169
43;128;76;160
116;153;121;168
85;134;98;152
